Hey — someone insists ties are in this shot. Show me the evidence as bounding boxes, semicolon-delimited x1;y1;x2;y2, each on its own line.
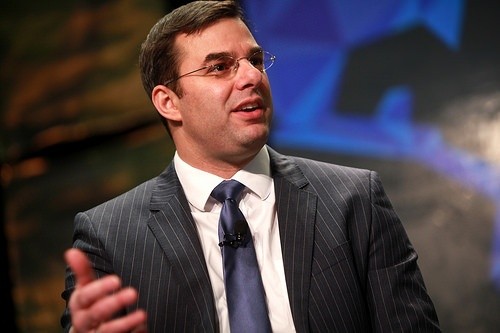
210;180;273;333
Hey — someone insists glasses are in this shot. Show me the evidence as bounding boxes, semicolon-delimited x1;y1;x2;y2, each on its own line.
163;51;276;86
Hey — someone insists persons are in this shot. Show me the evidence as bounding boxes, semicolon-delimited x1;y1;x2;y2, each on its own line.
61;0;443;333
